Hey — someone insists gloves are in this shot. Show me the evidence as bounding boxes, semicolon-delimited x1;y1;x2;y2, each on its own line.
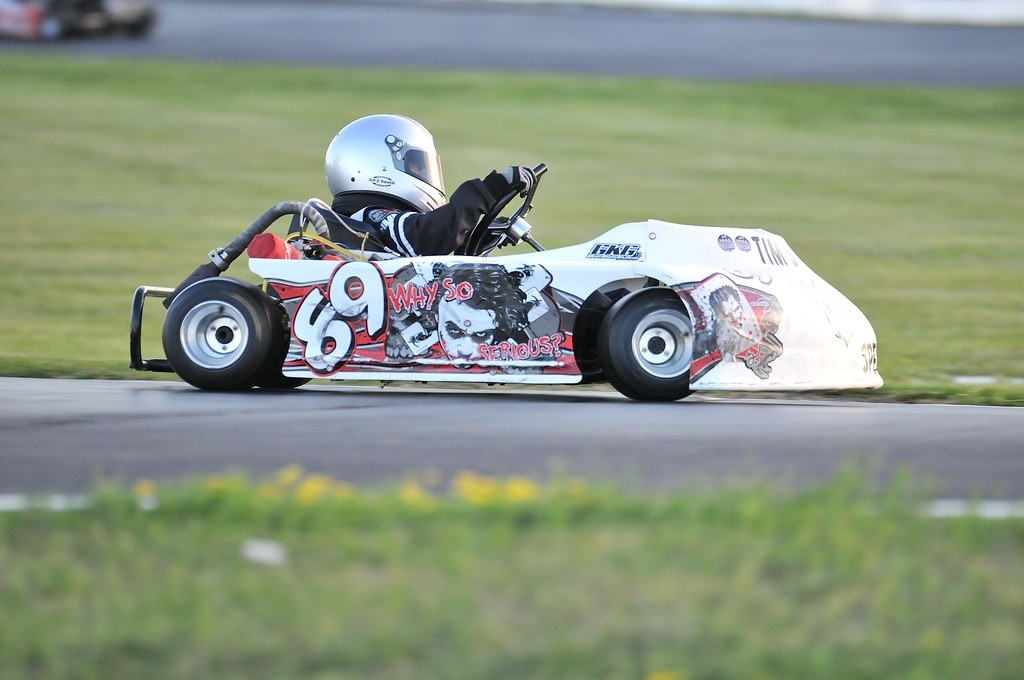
499;165;537;198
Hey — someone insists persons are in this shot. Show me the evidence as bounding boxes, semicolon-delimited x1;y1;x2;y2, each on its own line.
325;114;537;257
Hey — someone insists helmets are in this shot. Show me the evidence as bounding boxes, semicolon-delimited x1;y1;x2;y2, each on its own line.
324;114;446;215
